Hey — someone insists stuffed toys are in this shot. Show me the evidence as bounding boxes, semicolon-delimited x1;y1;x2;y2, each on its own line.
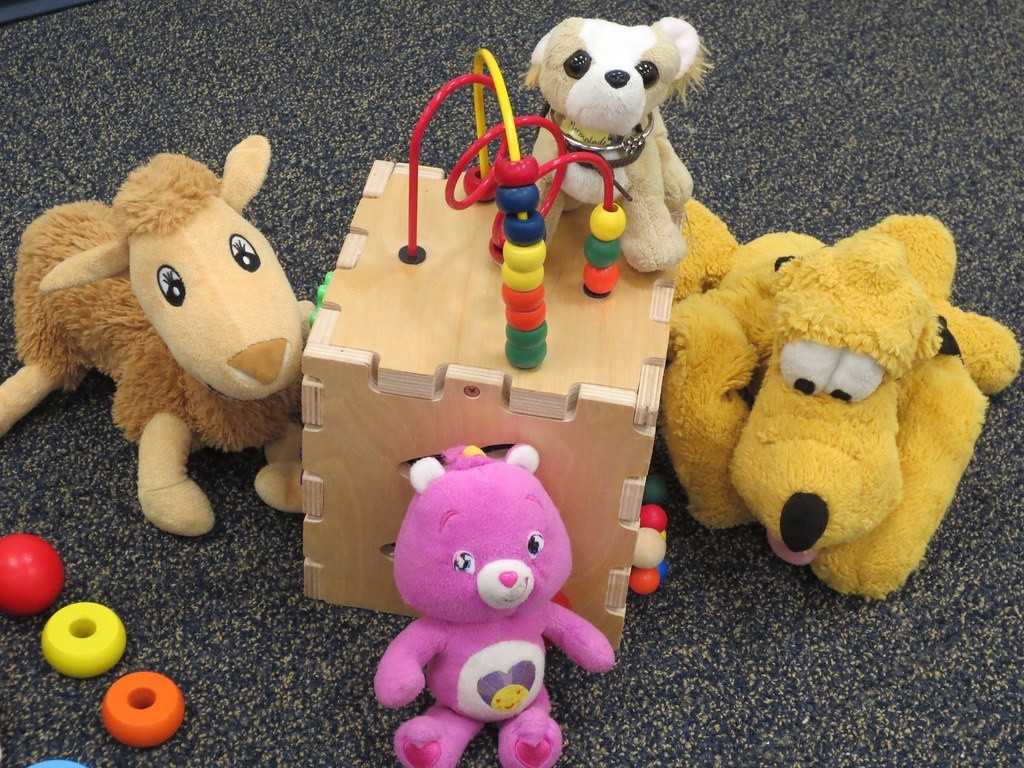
520;15;709;272
0;136;320;535
664;197;1021;599
373;442;615;767
